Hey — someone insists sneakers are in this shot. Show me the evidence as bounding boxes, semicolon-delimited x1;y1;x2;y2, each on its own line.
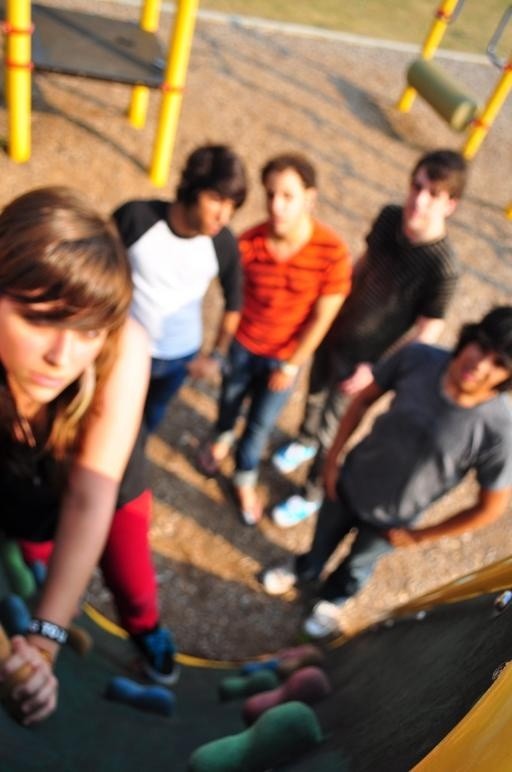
271;494;322;528
199;439;231;476
138;625;181;687
231;478;264;528
270;439;317;475
302;597;353;641
259;559;298;596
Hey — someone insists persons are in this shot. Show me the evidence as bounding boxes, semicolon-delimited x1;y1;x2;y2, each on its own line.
106;141;247;452
0;184;184;726
194;152;353;527
270;148;469;534
260;304;511;641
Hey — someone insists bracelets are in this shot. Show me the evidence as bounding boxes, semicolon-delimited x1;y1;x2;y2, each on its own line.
370;362;383;380
280;363;298;376
27;617;72;646
209;347;229;360
